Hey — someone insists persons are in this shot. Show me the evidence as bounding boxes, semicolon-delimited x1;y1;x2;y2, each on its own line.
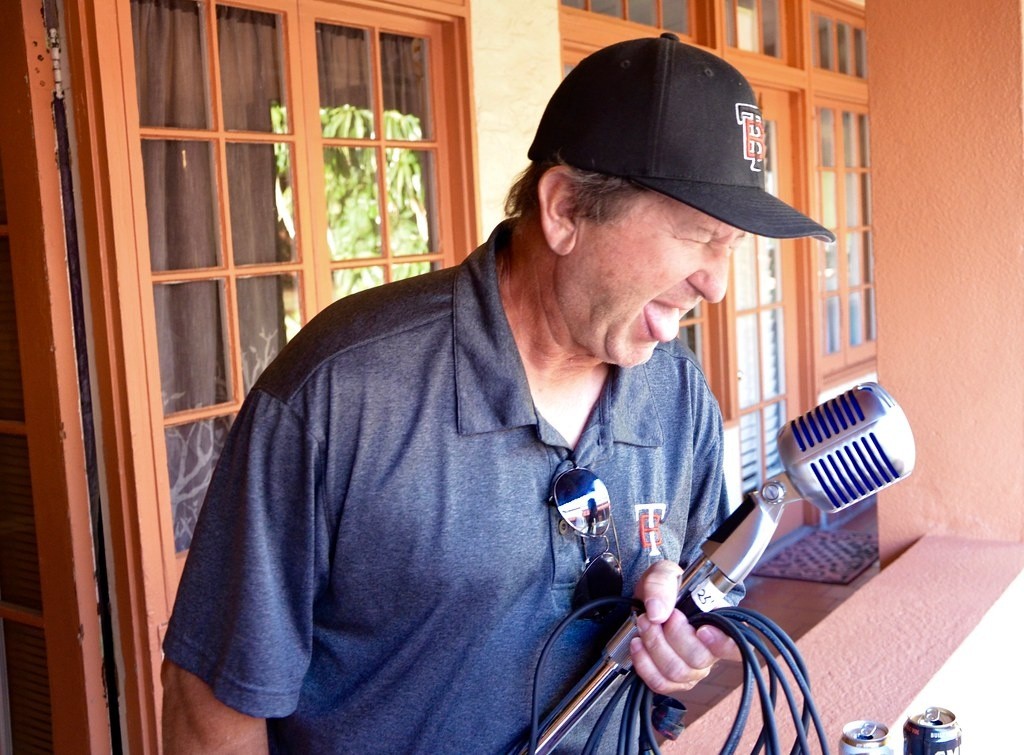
160;32;836;754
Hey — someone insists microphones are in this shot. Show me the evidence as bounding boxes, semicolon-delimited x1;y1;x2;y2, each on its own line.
671;383;918;621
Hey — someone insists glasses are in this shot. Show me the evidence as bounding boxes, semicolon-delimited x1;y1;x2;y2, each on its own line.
553;464;630;625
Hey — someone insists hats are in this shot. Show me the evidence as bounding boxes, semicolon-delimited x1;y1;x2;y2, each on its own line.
524;29;844;247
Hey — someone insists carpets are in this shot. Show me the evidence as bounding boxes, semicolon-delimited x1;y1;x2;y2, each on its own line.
754;526;879;585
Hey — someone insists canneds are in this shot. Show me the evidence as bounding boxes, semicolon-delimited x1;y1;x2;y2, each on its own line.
903;706;962;755
839;720;894;755
